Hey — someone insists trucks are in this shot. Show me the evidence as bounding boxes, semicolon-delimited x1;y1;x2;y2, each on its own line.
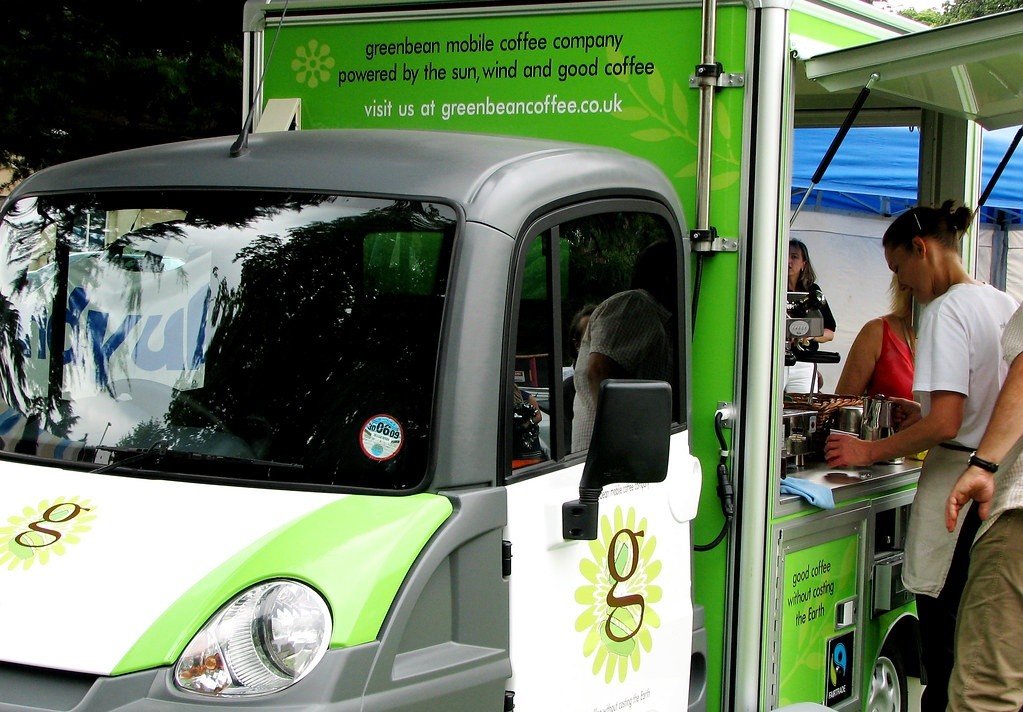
0;0;1023;711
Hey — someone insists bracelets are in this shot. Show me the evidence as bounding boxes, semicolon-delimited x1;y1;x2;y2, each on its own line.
812;337;815;340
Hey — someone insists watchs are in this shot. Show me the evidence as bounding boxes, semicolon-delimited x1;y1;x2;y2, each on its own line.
968;451;999;473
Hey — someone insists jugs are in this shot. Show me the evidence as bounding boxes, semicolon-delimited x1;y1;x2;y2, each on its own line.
859;393;908;442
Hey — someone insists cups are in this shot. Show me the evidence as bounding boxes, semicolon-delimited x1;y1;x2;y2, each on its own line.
838;406;863;438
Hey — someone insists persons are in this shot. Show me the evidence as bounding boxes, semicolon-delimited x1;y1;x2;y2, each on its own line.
834;274;917;400
782;240;837;393
823;198;1023;712
514;240;679;454
945;296;1023;712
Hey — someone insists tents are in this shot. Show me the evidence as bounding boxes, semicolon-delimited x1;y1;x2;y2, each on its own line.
791;125;1023;394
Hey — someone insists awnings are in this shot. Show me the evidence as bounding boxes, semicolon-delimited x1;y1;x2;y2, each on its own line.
788;7;1023;228
